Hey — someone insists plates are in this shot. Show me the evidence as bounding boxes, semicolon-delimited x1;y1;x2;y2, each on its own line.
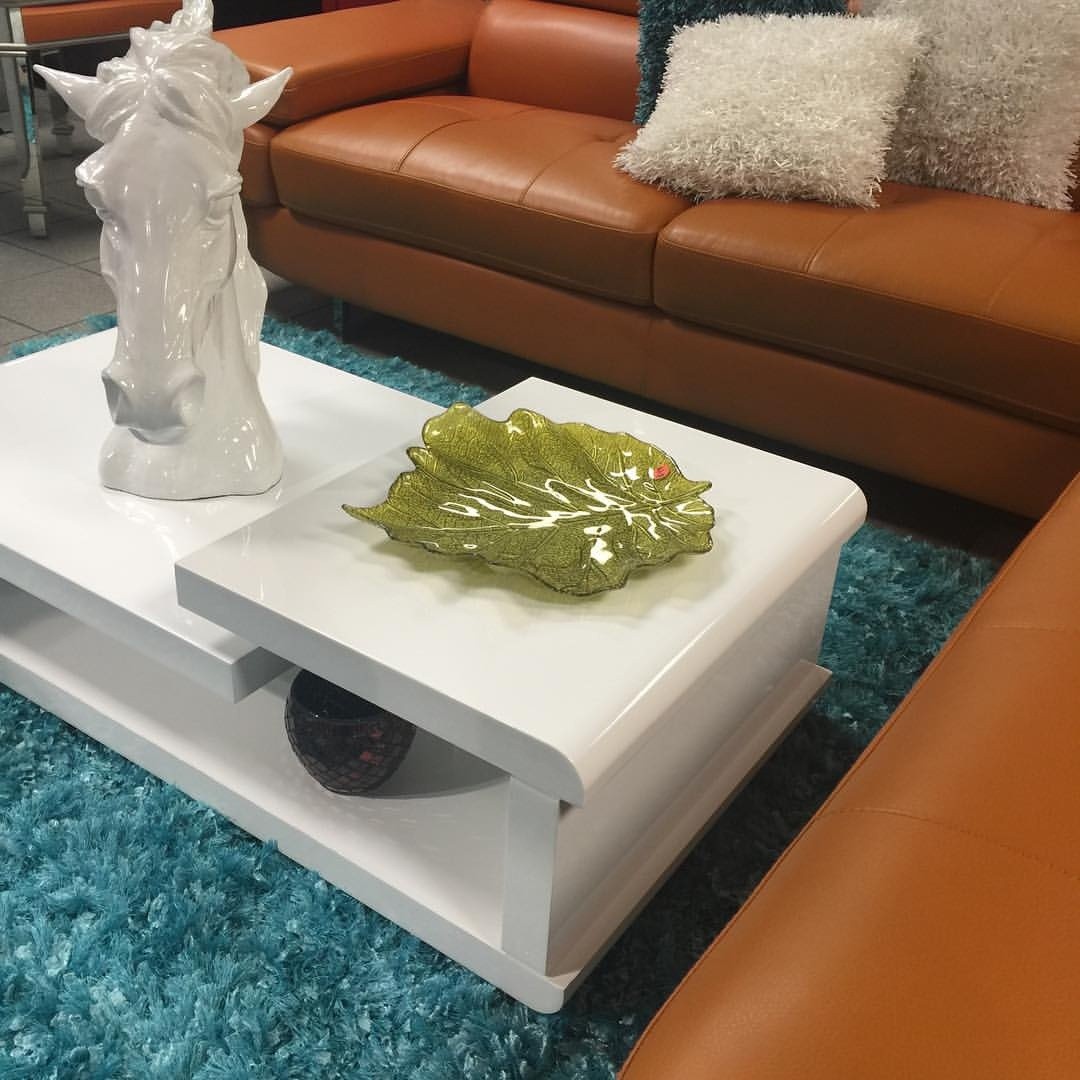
341;403;717;597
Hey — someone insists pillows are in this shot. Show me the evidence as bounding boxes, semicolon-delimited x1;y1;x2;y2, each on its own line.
614;10;924;211
871;0;1080;212
631;0;848;134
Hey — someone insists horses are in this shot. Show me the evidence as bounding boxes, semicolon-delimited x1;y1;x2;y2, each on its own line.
34;0;294;503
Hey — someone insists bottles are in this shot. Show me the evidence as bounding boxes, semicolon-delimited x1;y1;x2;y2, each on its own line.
284;670;417;795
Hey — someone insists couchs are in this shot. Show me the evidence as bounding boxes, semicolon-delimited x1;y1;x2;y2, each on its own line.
211;0;1079;518
617;472;1080;1079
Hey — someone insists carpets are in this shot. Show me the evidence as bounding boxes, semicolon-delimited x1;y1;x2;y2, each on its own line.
0;303;1005;1080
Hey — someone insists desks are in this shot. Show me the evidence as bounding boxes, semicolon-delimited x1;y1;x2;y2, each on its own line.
4;0;183;237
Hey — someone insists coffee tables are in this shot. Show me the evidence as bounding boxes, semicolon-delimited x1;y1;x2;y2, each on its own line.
2;326;867;1012
